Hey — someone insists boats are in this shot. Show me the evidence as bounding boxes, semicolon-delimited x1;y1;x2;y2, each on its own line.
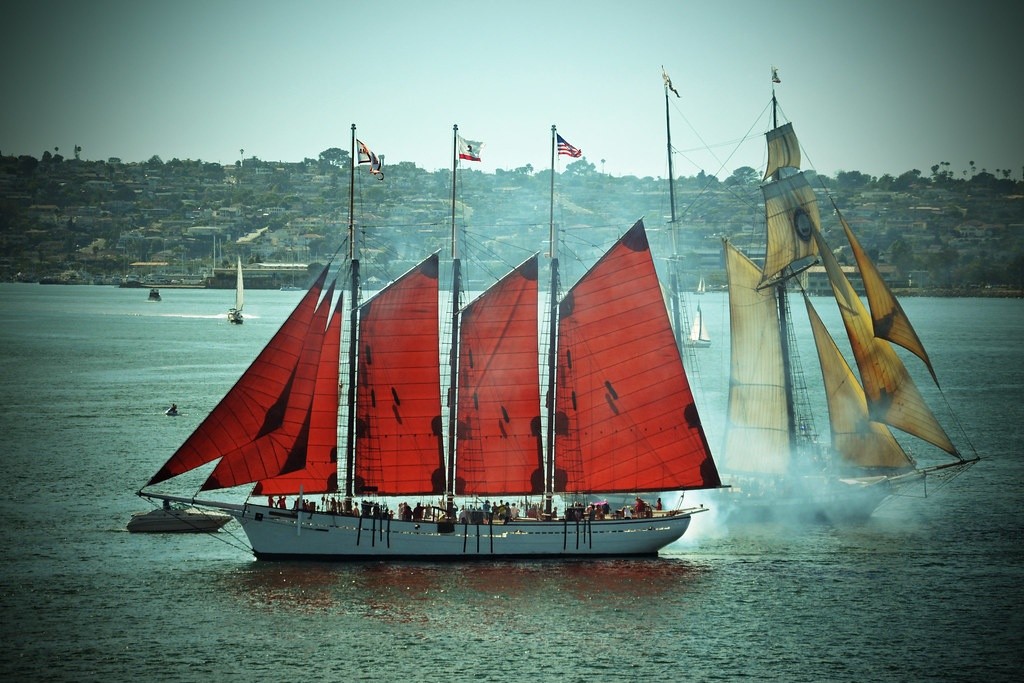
128;502;234;534
148;289;162;302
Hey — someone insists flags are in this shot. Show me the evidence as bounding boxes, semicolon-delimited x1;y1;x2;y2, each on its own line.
557;133;581;158
662;71;681;99
458;134;487;162
772;66;781;83
356;138;385;181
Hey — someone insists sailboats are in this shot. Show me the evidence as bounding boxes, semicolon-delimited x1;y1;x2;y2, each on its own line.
684;301;712;348
561;64;990;527
134;123;733;562
694;274;706;294
227;255;245;325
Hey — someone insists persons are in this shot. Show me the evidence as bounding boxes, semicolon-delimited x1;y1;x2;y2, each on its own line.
169;403;177;412
265;496;662;521
